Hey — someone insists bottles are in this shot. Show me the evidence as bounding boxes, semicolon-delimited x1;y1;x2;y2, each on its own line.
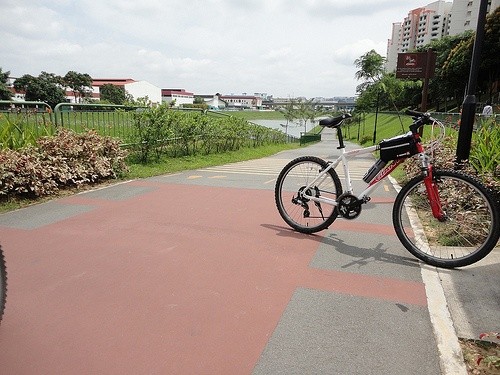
362;158;390;184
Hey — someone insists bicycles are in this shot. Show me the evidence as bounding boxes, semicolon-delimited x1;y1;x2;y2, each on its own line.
274;108;500;268
